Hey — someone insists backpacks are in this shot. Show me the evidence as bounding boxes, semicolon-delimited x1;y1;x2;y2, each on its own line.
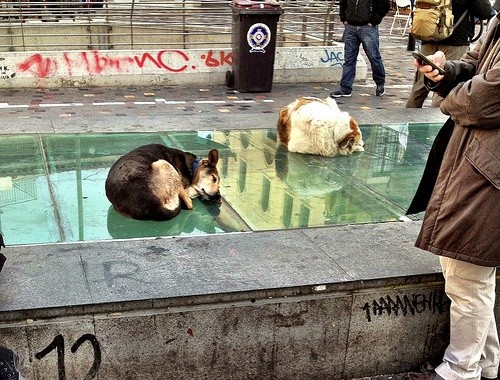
411;0;467;43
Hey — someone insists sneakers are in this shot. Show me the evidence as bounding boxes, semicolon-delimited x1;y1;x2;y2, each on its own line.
330;89;352;98
375;85;385;96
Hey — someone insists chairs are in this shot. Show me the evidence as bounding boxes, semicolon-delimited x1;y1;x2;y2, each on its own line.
389;0;413;36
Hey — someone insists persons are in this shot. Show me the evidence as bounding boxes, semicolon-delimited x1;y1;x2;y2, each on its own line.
406;0;493;108
330;0;390;97
405;0;500;380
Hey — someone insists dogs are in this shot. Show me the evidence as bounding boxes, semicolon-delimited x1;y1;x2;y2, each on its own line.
104;142;223;223
275;94;367;158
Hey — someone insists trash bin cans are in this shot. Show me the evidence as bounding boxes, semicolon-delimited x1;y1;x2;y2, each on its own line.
226;1;285;93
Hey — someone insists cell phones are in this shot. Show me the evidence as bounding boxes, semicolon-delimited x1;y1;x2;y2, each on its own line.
413;51;446;75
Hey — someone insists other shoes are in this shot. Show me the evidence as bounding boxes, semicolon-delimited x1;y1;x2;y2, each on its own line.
402;369;443;380
